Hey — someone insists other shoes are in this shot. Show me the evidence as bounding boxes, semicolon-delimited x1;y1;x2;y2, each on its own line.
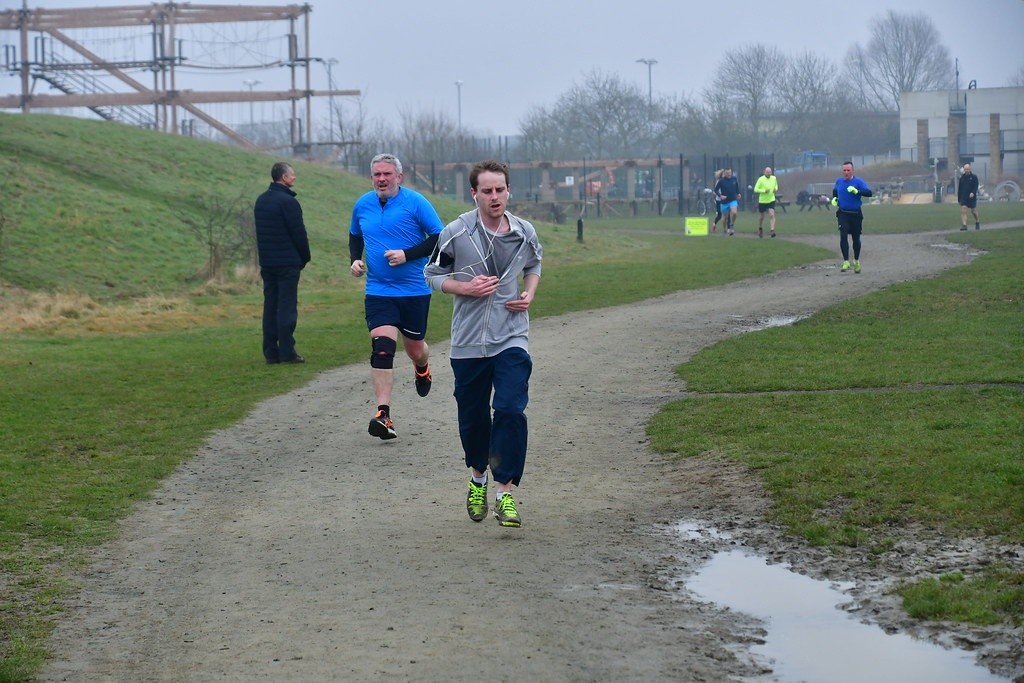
770;230;776;237
282;356;303;363
960;225;967;230
713;223;716;232
727;229;734;235
976;222;979;229
758;229;763;238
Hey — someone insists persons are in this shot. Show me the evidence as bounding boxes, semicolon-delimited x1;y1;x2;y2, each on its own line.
348;154;445;441
957;164;980;231
714;167;741;234
753;166;779;238
254;162;311;365
831;161;873;273
423;159;543;529
711;168;734;236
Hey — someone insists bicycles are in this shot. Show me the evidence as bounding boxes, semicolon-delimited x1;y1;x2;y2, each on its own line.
697;187;718;216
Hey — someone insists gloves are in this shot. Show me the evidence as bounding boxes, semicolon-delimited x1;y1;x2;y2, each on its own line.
832;197;838;206
847;186;858;195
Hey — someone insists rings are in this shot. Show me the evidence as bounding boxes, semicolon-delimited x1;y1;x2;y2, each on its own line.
393;259;396;262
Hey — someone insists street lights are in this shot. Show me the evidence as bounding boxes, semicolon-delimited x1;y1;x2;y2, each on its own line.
637;59;658;119
244;80;261;125
455;80;463;126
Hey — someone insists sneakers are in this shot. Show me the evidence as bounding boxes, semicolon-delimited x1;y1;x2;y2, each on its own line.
493;492;521;528
853;259;861;273
367;410;397;440
842;261;849;271
466;473;488;521
414;365;432;397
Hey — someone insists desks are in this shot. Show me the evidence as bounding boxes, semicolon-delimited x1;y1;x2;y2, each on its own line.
751;194;786;213
795;193;832;212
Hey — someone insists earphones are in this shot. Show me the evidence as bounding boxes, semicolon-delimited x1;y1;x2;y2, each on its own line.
507;192;509;199
474;194;477;203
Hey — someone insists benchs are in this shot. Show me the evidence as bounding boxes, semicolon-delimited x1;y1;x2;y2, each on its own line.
812;201;831;205
775;201;791;207
